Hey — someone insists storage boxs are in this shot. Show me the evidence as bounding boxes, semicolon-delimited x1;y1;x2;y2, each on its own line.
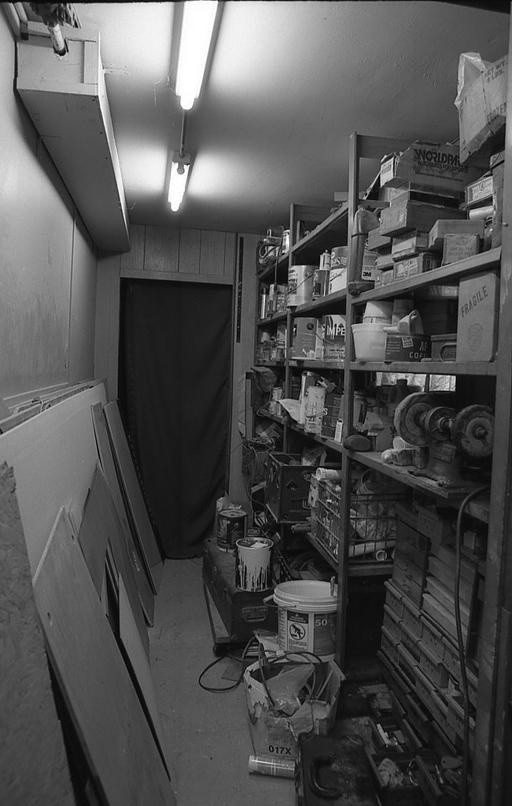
266;453;318;524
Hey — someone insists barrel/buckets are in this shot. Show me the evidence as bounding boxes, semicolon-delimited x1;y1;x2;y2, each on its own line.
217;509;248;553
235;537;275;592
273;580;338;663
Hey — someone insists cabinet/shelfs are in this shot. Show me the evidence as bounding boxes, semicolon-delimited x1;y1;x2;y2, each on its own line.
245;135;512;806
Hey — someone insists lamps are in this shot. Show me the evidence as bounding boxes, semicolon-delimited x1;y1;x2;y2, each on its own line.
160;0;225;213
